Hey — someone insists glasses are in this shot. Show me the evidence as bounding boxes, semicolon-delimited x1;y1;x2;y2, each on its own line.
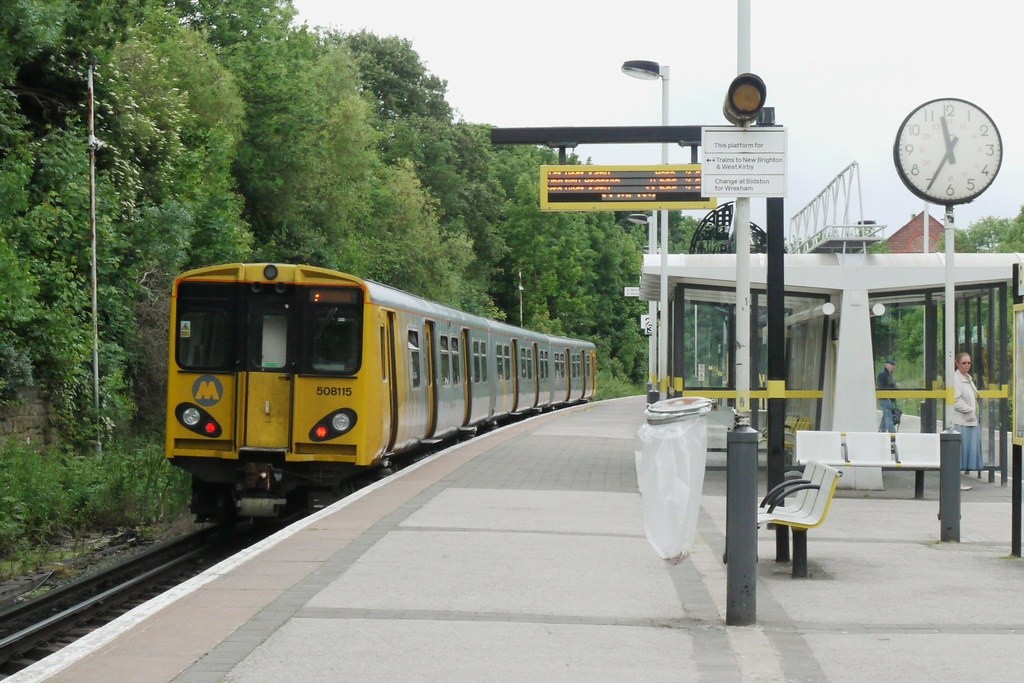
962;362;970;364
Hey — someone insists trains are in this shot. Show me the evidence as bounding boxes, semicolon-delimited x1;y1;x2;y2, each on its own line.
164;258;597;527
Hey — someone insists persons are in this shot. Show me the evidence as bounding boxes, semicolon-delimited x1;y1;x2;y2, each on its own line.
953;350;985;493
876;357;900;432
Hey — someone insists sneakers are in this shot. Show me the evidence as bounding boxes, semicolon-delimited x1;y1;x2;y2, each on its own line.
961;482;972;491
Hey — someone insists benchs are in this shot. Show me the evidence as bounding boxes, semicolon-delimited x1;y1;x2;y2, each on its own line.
758;457;844;579
792;428;941;500
756;414;812;459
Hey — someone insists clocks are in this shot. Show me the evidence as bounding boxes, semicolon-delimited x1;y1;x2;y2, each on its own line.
892;98;1004;207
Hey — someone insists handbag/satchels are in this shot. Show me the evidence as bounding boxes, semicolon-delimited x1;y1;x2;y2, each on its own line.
892;407;902;425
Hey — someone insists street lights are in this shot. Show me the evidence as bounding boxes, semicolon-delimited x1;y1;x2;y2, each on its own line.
626;211;658;392
620;58;671;403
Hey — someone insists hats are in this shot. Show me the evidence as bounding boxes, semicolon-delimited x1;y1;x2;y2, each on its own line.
886;360;896;364
